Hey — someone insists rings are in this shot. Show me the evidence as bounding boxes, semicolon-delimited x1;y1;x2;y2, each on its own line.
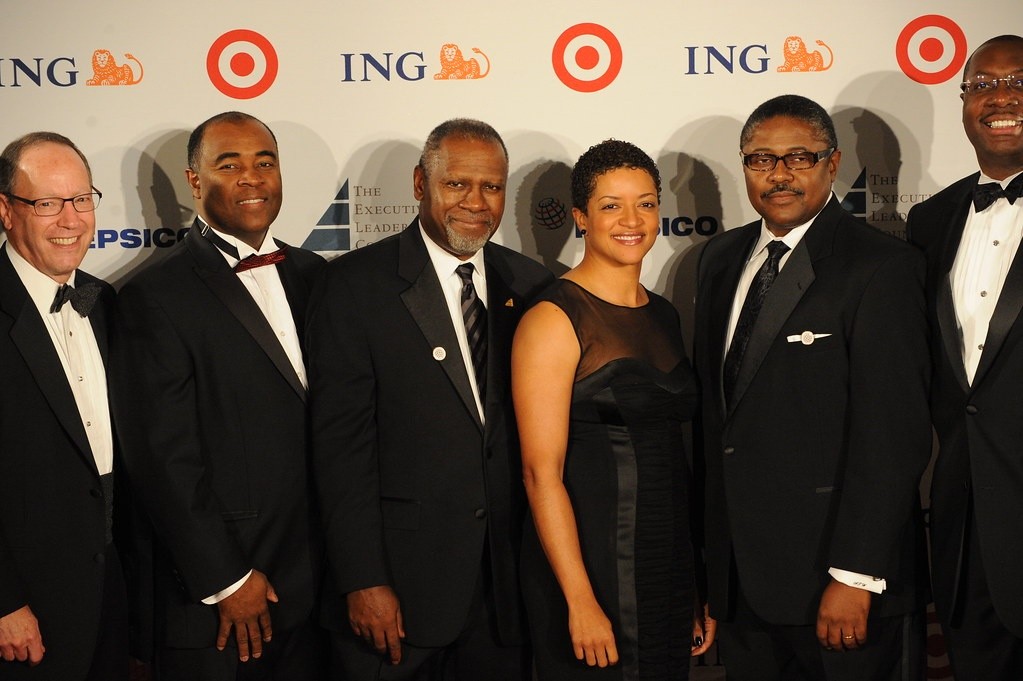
843;634;855;639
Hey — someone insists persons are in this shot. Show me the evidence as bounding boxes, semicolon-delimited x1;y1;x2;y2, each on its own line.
0;131;117;681
108;111;330;681
512;138;717;681
906;34;1023;681
692;95;933;681
305;118;555;681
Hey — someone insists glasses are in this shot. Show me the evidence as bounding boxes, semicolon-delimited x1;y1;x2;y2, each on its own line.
0;185;101;216
959;72;1023;92
739;148;834;172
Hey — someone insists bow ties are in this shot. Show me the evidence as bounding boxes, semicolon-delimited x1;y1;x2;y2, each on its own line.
49;280;101;318
195;222;287;272
972;172;1023;212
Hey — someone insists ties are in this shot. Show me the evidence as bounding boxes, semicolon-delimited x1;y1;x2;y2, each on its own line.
454;262;490;416
722;241;790;395
